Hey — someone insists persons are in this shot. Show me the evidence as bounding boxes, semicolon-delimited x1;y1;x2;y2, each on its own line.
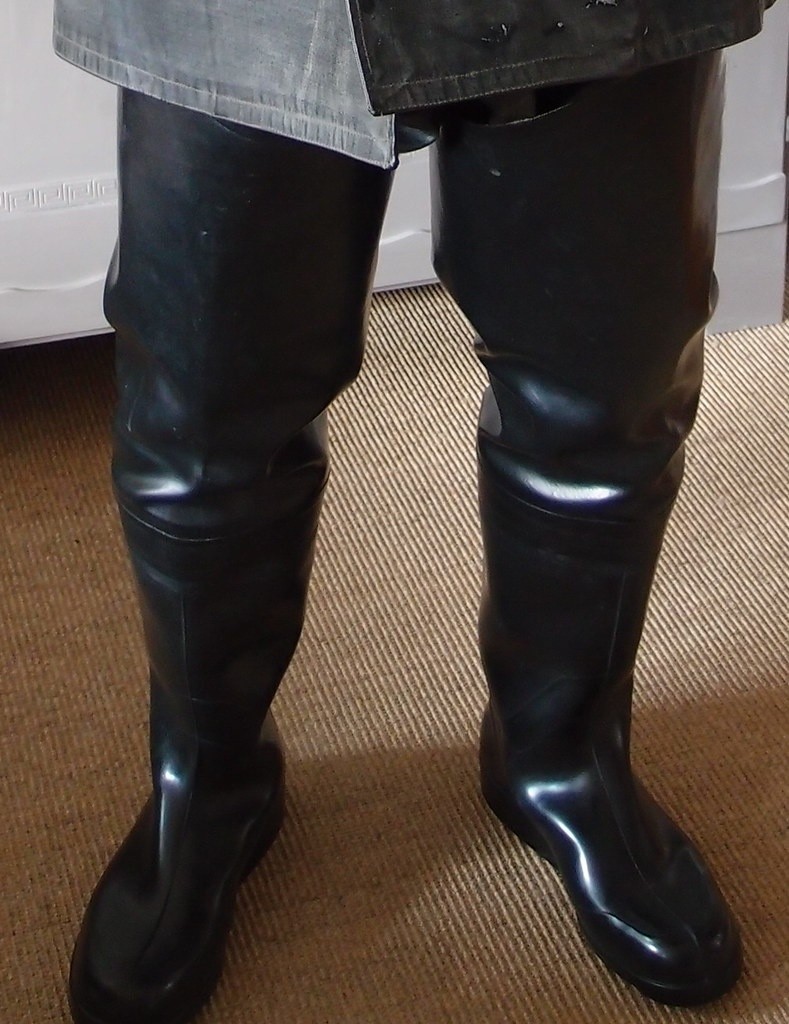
53;0;776;1024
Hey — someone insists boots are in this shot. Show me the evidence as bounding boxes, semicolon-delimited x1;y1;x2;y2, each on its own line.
67;465;332;1024
476;443;741;1005
